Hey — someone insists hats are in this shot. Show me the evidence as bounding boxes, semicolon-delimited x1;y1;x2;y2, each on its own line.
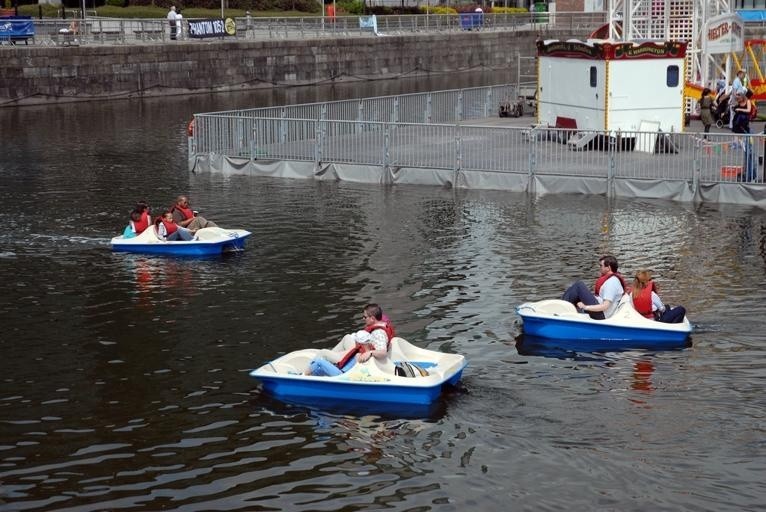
350;329;372;345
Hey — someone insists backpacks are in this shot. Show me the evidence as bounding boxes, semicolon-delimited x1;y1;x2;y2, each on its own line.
394;361;430;377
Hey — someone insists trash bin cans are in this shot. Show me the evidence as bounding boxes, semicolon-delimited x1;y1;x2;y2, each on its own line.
0;15;36;46
460;7;484;31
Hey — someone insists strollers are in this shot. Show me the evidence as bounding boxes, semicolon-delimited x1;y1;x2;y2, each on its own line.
712;97;731;129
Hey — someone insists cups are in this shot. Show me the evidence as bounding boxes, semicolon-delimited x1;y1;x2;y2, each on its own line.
192;211;198;219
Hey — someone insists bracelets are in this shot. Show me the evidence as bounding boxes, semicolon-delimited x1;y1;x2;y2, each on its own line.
370;350;372;355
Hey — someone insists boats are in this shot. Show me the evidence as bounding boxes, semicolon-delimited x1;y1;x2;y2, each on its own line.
250;336;469;417
518;296;692;343
513;335;687;357
113;226;256;258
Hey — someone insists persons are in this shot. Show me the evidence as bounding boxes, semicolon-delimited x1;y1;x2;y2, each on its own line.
631;270;686;323
695;68;752;141
167;6;182;39
468;8;483;31
123;195;218;240
305;330;372;376
562;256;627;320
318;303;393;363
